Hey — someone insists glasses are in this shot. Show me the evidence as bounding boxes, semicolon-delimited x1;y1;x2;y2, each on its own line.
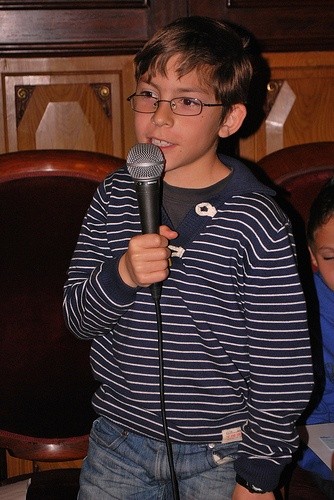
125;92;230;116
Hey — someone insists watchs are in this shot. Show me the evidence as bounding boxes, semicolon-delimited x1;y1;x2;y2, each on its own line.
236;472;272;494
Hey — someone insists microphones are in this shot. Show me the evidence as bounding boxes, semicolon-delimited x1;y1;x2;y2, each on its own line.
126;143;166;301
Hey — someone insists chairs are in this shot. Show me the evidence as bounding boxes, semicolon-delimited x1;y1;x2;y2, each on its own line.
0;149;128;500
255;140;334;500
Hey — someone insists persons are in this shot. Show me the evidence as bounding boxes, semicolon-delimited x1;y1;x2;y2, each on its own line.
62;15;315;500
274;177;334;500
